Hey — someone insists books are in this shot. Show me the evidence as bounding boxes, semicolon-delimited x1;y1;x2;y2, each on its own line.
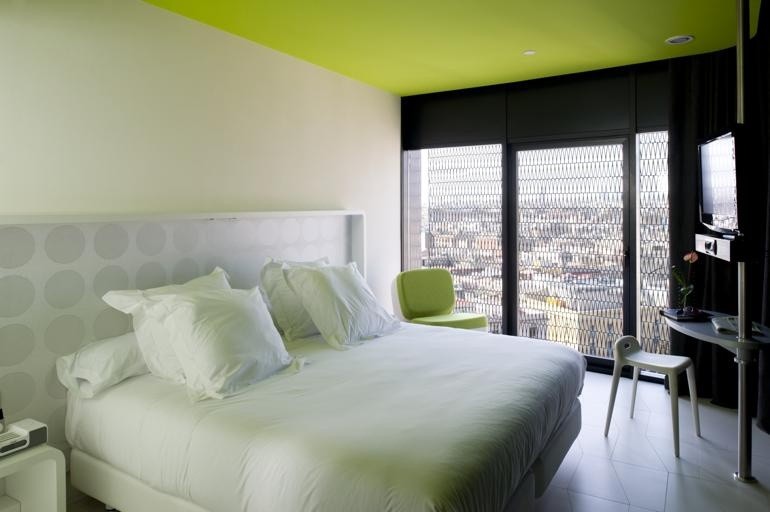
659;308;715;323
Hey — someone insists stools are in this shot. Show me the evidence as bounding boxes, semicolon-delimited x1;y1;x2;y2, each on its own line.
602;335;702;459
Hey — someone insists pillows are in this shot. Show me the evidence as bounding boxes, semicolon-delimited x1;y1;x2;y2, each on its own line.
134;283;312;402
258;257;336;342
101;265;234;386
279;260;398;352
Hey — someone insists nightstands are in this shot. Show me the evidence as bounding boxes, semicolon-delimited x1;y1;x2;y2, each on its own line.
0;444;68;512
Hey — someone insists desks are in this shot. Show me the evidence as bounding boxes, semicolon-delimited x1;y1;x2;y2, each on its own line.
659;305;768;486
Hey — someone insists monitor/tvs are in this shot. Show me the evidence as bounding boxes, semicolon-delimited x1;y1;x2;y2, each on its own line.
697;123;748;239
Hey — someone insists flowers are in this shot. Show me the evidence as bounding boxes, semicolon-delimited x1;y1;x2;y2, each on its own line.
646;250;699;308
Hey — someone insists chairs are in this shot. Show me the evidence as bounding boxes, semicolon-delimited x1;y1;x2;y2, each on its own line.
399;266;489;331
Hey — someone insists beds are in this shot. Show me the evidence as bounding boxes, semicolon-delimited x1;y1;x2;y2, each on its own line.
62;312;587;510
